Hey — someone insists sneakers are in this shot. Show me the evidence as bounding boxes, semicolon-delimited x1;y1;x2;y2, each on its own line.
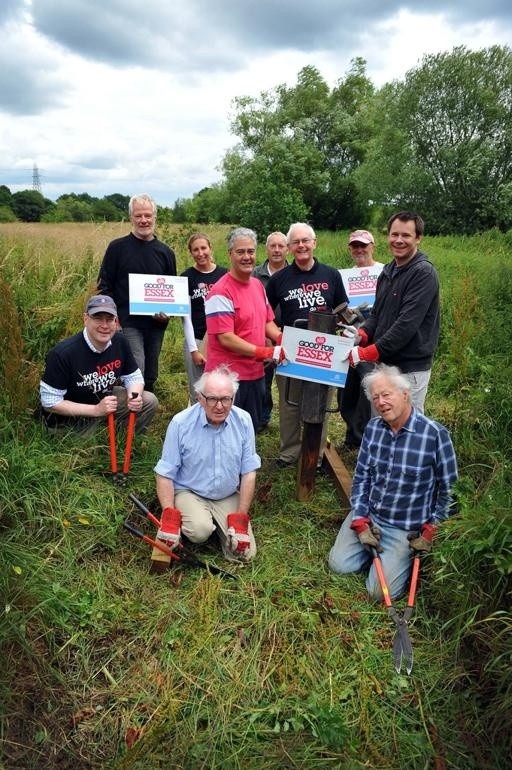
268;458;290;475
314;465;329;478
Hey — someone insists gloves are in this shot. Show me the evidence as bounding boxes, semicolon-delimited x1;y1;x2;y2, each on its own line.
407;524;438;560
342;327;368;346
255;334;290;367
226;513;251;557
349;518;384;553
349;344;381;369
157;507;181;549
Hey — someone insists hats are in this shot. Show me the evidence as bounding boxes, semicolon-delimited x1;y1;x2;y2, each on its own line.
347;230;375;247
87;295;118;319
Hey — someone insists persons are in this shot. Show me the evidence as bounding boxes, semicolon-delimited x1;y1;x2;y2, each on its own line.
153;363;262;565
265;221;353;477
330;363;461;602
335;230;384;455
35;295;159;453
97;193;178;392
342;211;441;418
180;227;290;435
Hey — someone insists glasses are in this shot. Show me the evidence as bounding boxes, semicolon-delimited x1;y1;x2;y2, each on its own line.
200;390;235;408
87;313;116;325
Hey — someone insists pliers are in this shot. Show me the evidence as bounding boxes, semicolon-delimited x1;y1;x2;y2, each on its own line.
123;493;237;581
368;522;422;676
104;391;139;489
